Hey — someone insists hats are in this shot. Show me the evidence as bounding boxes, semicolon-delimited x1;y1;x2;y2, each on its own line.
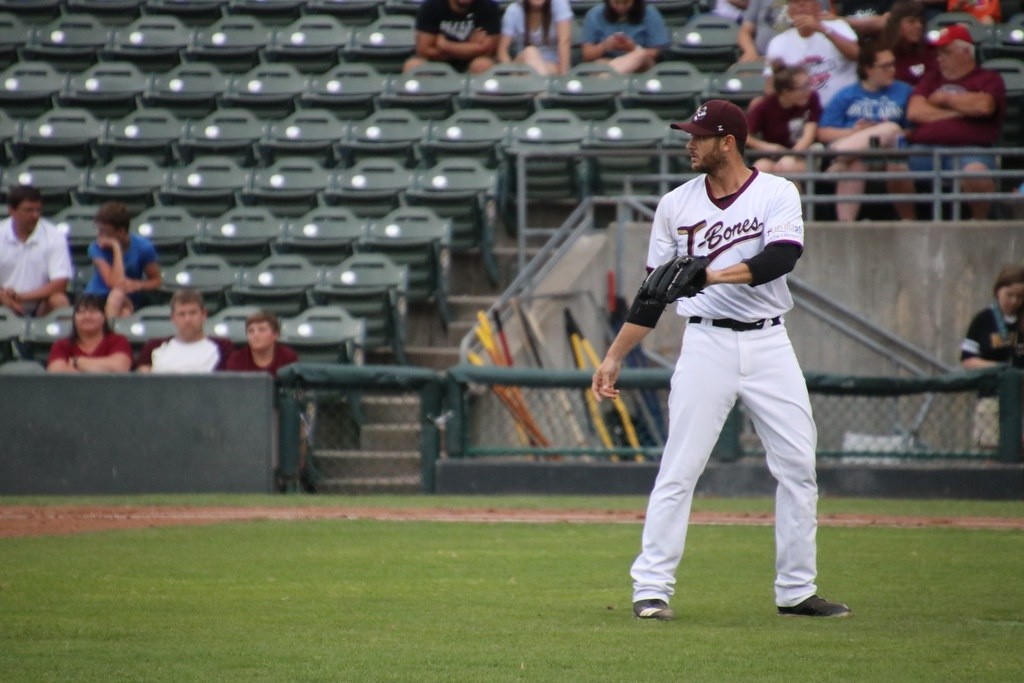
930;26;973;49
670;100;748;144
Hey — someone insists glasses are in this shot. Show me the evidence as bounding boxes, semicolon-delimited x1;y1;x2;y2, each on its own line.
872;61;897;72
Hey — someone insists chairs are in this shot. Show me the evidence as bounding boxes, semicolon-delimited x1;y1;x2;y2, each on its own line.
0;0;1024;450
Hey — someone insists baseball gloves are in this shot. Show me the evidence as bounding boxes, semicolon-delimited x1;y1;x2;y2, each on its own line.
643;255;710;304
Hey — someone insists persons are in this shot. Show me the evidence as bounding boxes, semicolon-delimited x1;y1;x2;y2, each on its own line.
134;285;227;375
46;295;134;372
0;186;76;319
83;202;163;326
958;266;1024;465
227;310;316;493
590;101;852;619
402;0;672;79
706;0;1007;222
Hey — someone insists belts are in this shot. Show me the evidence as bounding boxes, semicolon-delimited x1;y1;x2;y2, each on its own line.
689;316;781;332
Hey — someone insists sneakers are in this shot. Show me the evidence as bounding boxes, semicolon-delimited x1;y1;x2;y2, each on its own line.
634;599;674;621
777;594;852;618
